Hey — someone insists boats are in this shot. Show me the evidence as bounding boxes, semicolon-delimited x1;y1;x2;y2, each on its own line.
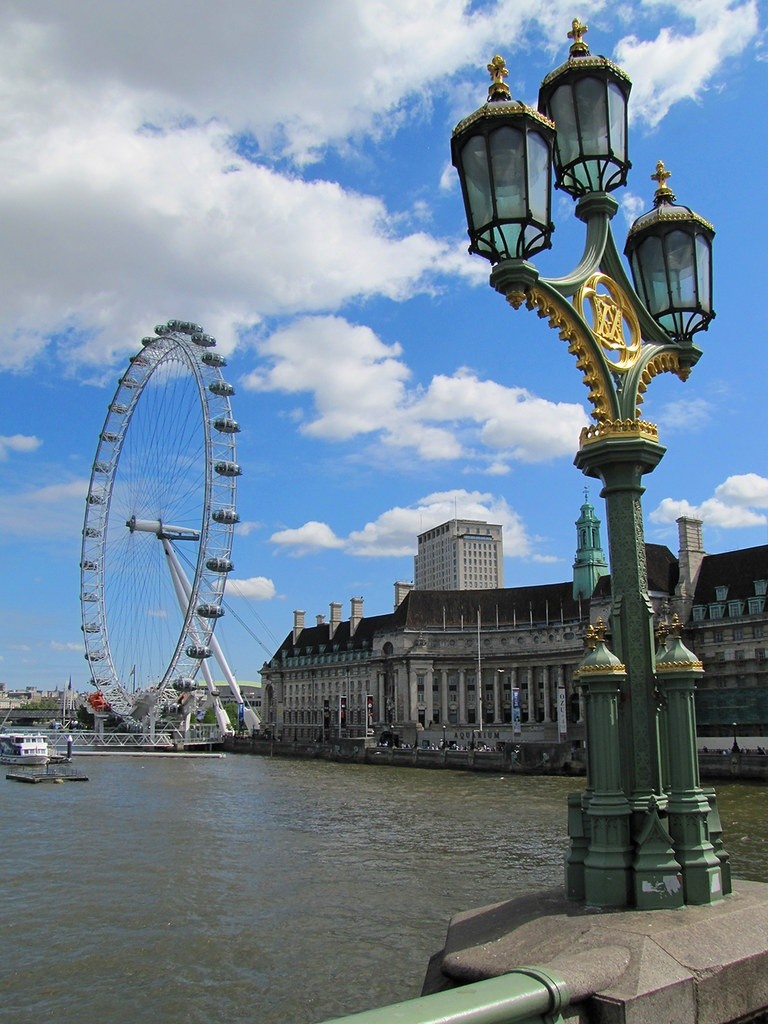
0;733;50;765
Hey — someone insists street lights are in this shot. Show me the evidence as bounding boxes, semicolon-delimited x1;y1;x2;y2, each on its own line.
390;725;395;749
445;17;734;913
442;725;445;752
